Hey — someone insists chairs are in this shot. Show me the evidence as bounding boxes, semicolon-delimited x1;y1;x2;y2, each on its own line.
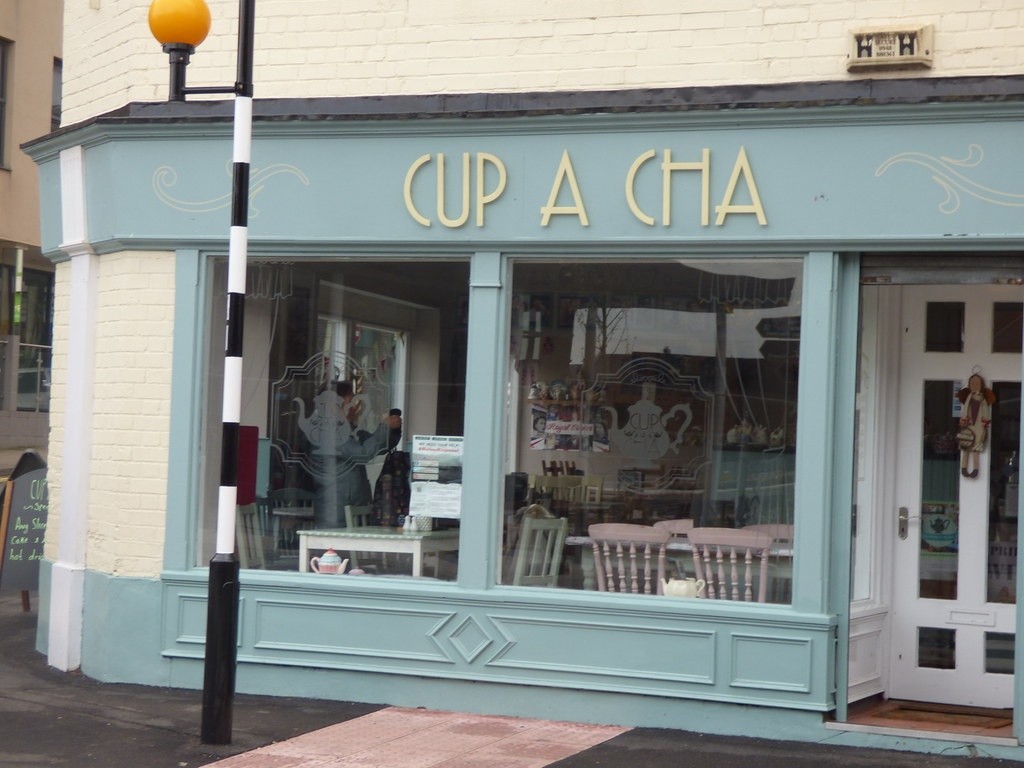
238;458;797;604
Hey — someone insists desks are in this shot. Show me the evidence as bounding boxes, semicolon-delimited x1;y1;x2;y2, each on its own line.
295;527;459;577
625;488;703;501
525;497;613;514
263;507;315;550
564;535;794;591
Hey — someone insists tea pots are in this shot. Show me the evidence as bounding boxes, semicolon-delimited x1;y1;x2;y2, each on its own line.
727;417;782;446
929;516;950;534
310;548;350;575
658;577;706;599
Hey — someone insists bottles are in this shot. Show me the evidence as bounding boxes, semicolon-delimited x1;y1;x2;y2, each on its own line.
402;515;418;532
997;457;1019;522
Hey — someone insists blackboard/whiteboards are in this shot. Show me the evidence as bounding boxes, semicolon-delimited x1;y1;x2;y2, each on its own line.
0;468;46;591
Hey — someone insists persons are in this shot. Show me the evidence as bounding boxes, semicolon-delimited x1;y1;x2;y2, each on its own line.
310;380;402;572
531;416;549;440
593;422;606;443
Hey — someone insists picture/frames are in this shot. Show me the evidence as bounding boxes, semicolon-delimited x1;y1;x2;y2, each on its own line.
451;288;690;331
